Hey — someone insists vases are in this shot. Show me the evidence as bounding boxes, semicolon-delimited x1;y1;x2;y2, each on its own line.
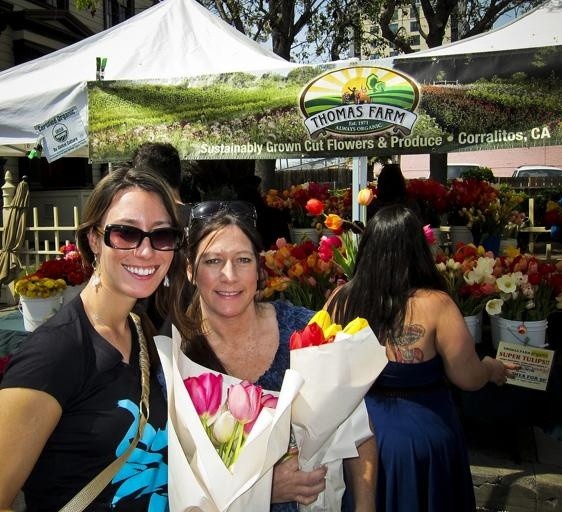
429;226;474;260
19;282;87;332
289;227;320;247
462;310;548;350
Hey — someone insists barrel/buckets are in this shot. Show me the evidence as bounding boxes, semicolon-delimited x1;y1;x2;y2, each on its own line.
447;226;472;250
495;316;549;348
321;226;332;236
16;295;64;332
431;228;438;245
465;313;485;342
291;228;317;244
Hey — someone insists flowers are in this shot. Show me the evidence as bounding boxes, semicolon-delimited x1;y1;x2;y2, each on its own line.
12;244;83;298
404;178;529;243
265;181;330;227
256;235;357;311
433;241;562;321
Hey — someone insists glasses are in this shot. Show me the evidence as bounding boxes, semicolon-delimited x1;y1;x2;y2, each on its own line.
91;223;184;252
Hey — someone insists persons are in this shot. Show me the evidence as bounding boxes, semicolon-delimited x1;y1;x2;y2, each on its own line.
232;174;295;248
166;194;382;511
367;162;416;217
134;137;187;329
0;163;210;511
319;198;523;512
